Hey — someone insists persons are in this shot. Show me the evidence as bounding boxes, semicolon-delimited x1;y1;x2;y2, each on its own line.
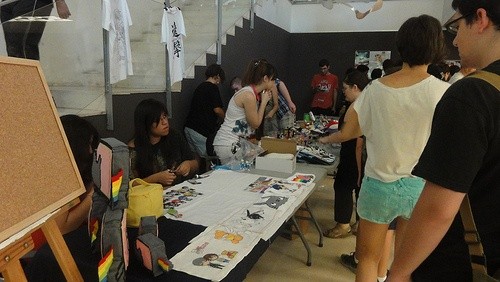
0;0;71;60
318;68;396;282
126;98;201;186
311;60;339;116
189;57;296;166
343;14;451;282
344;59;476;85
384;0;500;282
45;114;101;236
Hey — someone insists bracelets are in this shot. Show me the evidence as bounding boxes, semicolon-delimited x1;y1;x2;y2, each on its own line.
329;135;332;144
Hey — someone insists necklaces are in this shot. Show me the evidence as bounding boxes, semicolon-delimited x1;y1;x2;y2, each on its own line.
249;83;260;101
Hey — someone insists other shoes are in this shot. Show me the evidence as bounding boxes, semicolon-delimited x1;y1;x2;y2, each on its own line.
340;252;358;274
323;222;358;239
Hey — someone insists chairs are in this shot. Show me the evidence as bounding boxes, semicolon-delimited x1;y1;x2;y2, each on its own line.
204;133;220;171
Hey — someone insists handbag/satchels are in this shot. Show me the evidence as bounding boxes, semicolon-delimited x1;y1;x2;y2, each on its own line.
127;177;164;228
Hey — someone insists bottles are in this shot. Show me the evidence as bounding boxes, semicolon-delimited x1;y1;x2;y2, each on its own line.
303;113;310;124
230;143;253;171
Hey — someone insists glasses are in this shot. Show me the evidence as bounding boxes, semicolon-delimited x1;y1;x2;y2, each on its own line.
444;15;466;35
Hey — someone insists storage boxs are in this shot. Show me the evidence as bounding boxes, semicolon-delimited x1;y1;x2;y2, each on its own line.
290;206;309;240
255;136;301;173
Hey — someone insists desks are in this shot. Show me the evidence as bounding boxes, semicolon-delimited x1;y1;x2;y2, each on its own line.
28;130;340;282
